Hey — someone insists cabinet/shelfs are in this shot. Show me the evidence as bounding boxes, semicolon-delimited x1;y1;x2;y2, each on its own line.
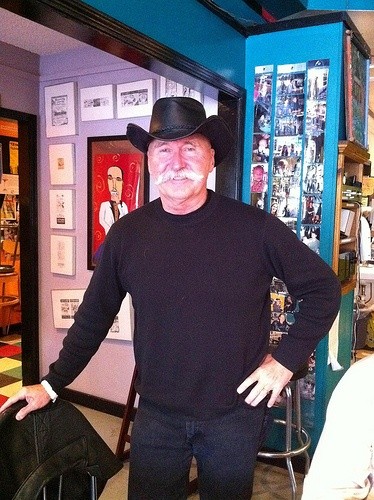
334;143;369;296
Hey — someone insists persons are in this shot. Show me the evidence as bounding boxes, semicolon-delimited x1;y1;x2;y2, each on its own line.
253;69;327;399
0;97;343;500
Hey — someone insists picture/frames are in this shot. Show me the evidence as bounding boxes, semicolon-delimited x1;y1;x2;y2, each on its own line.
344;29;371;151
87;134;150;270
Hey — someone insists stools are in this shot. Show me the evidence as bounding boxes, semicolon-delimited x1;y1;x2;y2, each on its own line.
0;265;19;338
258;372;318;500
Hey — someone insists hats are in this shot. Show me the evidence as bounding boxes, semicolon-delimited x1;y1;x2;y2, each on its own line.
125;94;235;167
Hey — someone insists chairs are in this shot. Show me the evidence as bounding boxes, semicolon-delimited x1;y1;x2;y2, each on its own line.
0;400;101;500
